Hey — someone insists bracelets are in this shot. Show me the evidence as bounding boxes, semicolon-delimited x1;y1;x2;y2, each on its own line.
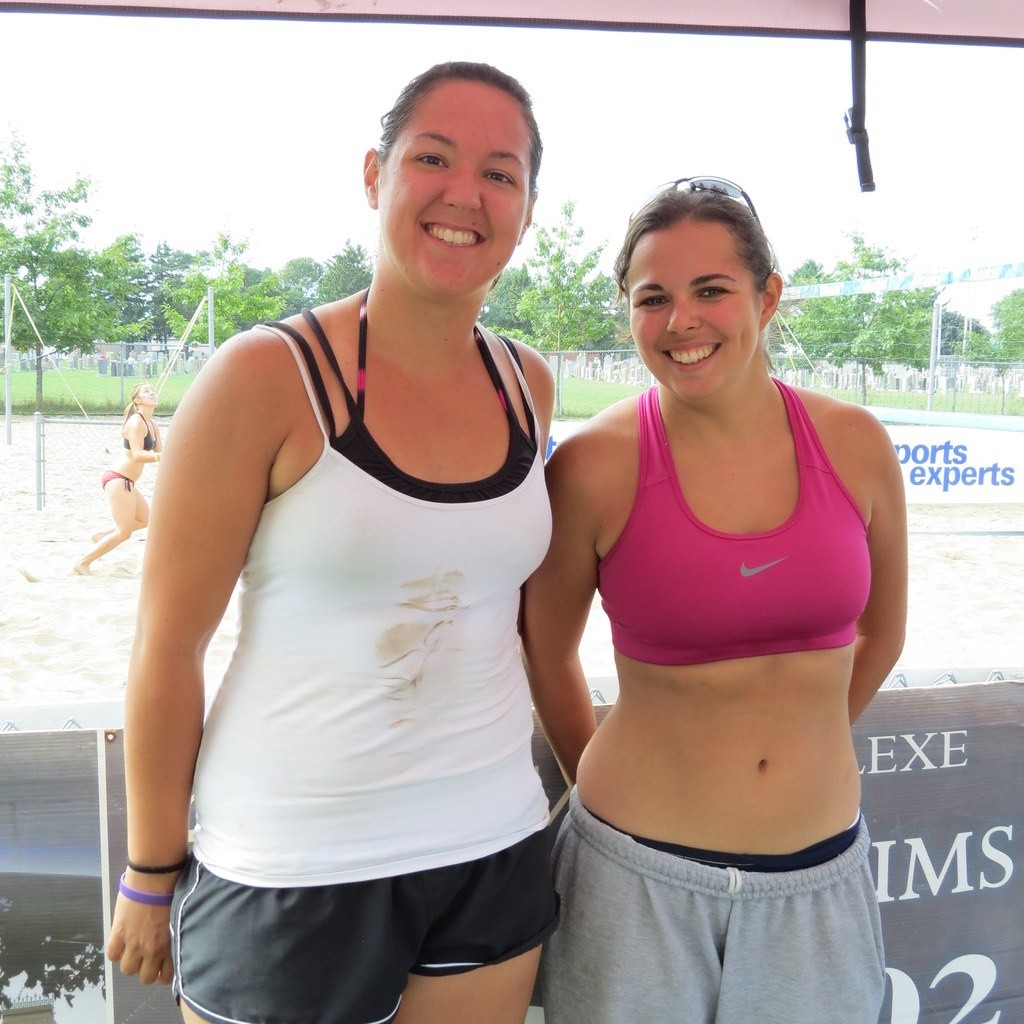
119;860;189;905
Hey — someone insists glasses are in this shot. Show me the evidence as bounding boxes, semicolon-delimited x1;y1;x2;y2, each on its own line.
628;176;766;238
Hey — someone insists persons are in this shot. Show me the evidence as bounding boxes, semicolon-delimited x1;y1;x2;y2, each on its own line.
70;383;163;576
107;63;557;1024
521;183;909;1024
